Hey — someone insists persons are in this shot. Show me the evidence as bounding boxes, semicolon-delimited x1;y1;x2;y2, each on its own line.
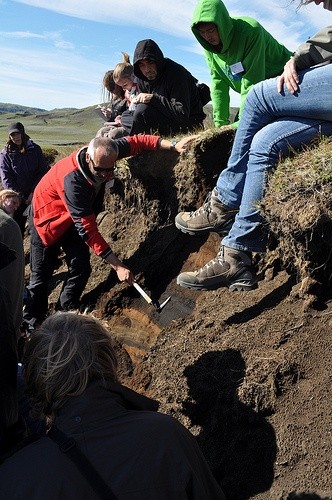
0;310;227;500
0;208;26;458
25;134;188;333
0;189;23;215
94;38;211;137
176;0;332;292
0;122;52;239
191;1;296;131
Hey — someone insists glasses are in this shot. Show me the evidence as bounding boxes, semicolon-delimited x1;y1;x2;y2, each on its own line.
90;157;116;173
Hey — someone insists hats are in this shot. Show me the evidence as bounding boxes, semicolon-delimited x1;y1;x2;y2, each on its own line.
8;122;22;135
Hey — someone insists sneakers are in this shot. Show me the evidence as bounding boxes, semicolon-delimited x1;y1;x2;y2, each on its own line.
174;186;239;238
176;246;258;291
